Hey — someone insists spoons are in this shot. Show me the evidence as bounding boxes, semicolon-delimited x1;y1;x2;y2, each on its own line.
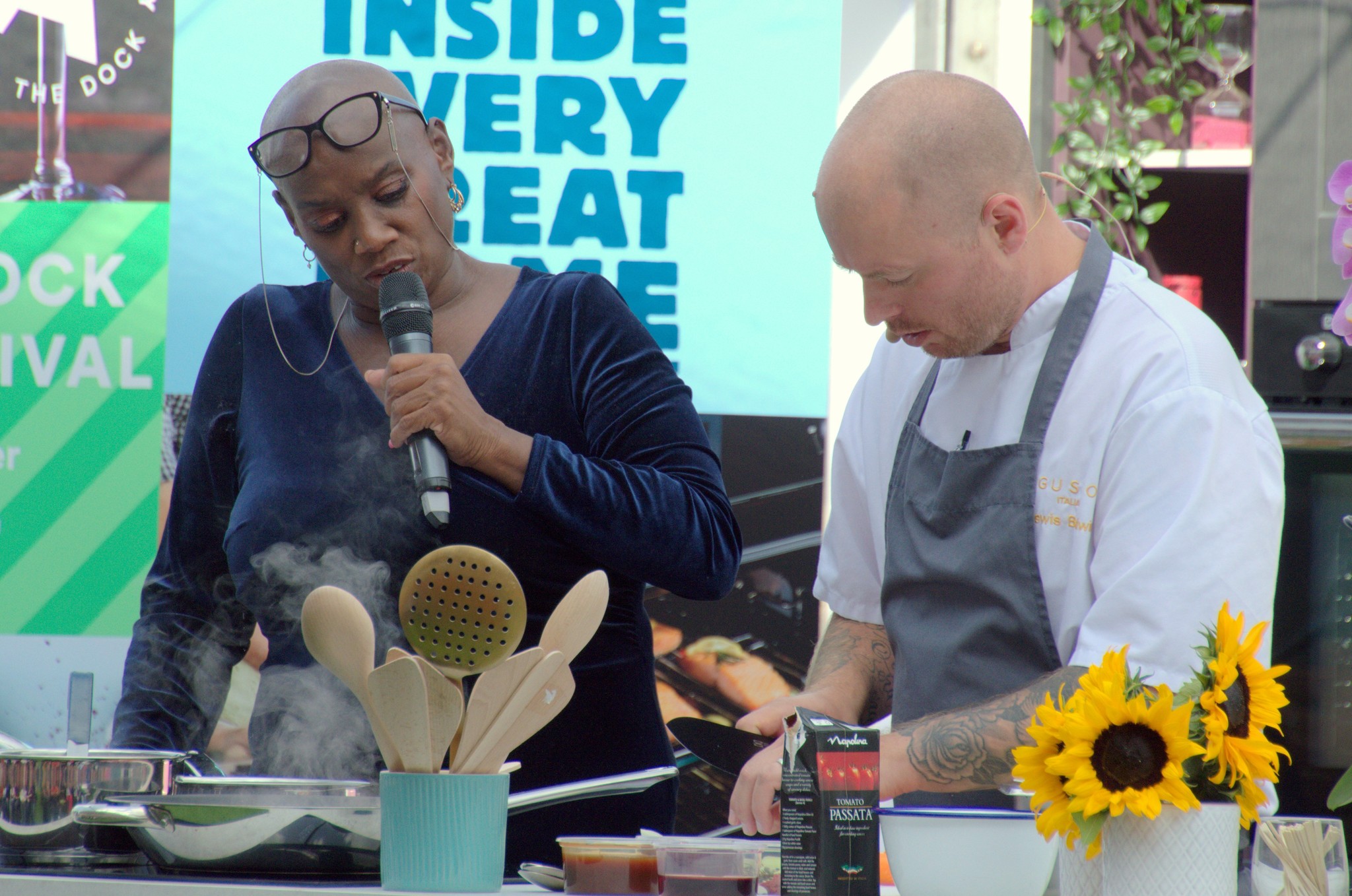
518;863;564;890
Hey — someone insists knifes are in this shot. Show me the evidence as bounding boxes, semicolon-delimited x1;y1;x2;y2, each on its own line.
666;716;775;779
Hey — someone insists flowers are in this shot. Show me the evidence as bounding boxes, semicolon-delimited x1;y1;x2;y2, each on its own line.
1011;601;1292;861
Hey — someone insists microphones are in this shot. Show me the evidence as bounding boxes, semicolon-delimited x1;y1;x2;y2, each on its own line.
885;328;901;343
379;272;453;530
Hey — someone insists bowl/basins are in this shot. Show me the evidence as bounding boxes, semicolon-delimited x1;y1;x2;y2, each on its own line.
555;836;660;896
653;837;764;896
176;774;370;796
873;806;1063;896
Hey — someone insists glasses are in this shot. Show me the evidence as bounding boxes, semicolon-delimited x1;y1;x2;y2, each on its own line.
246;91;428;179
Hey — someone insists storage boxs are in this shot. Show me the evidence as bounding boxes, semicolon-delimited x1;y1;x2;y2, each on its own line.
781;706;880;896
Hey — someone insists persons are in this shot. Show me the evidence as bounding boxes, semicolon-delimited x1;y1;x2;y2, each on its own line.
728;69;1286;836
105;58;745;880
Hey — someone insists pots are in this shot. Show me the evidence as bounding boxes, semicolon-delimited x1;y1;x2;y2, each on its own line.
71;767;680;882
0;748;199;869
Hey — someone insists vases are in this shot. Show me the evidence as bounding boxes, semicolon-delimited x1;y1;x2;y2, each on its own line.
1059;801;1241;896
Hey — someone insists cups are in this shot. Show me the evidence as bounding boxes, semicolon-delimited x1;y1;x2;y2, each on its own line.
1251;816;1349;896
380;769;510;892
